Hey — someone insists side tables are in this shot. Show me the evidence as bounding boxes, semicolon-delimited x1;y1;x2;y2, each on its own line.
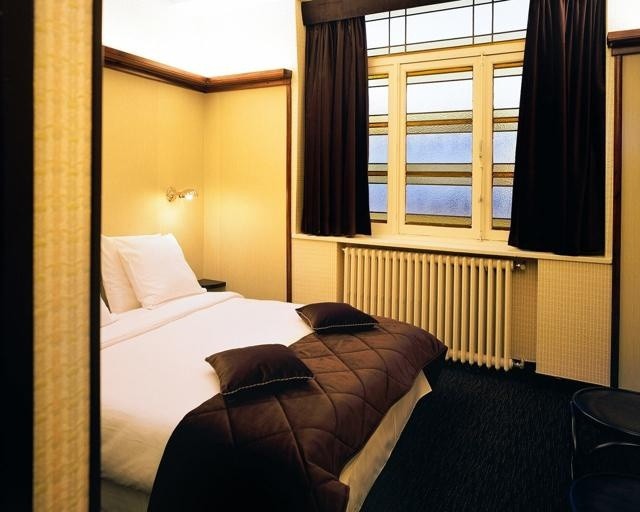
569;387;640;512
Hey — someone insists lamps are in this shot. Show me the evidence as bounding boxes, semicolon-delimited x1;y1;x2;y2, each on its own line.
165;186;198;203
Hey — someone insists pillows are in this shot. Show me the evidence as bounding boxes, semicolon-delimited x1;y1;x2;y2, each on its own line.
204;343;316;402
99;296;120;328
100;232;161;315
114;233;207;309
295;302;380;334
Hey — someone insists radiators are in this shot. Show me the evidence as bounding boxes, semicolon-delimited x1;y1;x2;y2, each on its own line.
339;246;526;372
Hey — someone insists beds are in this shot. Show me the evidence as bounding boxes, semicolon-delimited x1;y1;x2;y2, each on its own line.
99;292;448;512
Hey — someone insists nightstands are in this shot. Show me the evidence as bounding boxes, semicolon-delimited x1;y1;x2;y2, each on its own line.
196;278;226;292
